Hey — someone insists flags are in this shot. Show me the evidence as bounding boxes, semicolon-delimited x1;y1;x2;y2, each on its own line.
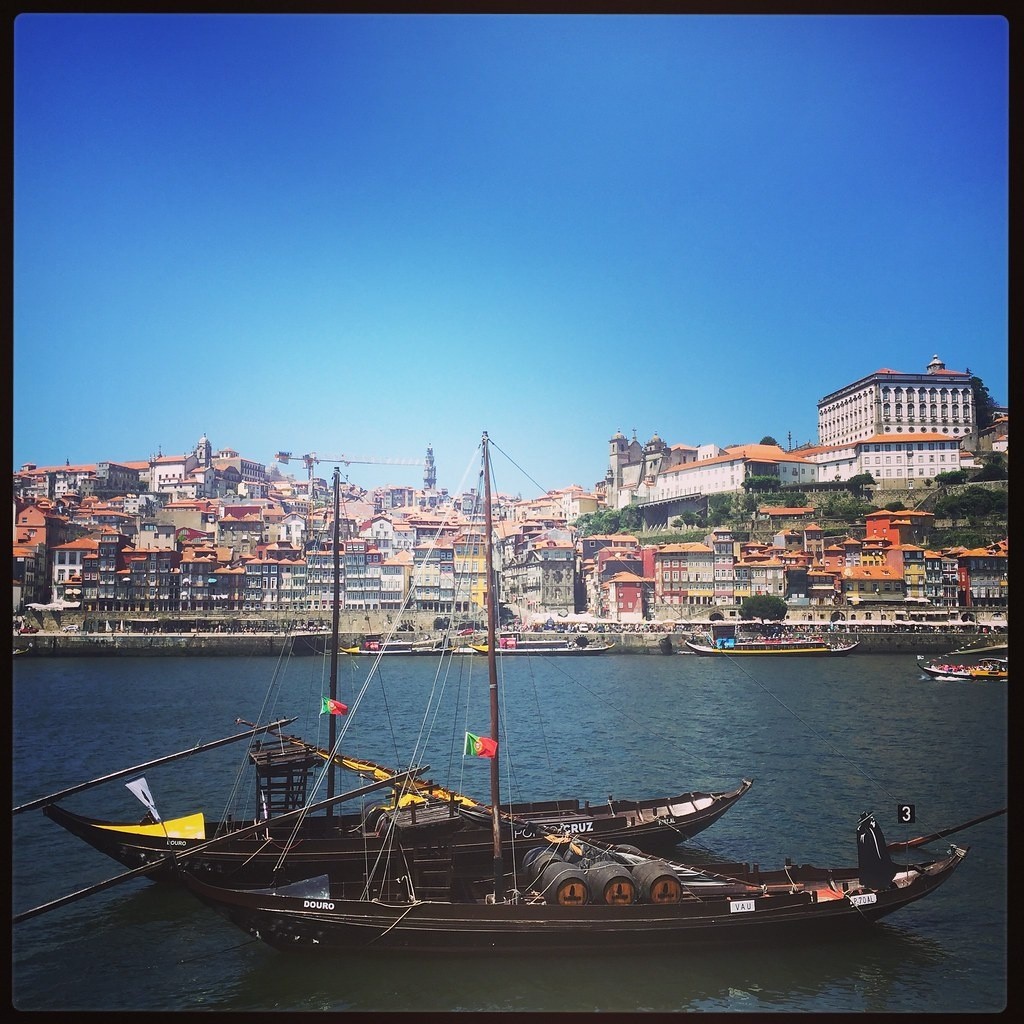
321;697;348;716
464;731;498;759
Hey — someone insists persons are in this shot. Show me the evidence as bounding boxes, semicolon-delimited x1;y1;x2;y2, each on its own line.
143;625;252;635
500;624;987;632
931;660;1002;673
826;641;853;650
855;811;898;891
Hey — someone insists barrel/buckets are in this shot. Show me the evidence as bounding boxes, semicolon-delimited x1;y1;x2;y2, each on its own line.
522;846;683;904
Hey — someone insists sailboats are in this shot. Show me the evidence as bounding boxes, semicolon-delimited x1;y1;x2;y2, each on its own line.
177;431;973;952
40;464;751;887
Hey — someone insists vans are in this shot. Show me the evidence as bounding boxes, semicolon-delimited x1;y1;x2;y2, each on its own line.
579;624;588;632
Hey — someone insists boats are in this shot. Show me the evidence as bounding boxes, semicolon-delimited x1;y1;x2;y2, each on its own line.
685;633;861;657
340;634;457;657
470;635;615;655
916;658;1009;681
